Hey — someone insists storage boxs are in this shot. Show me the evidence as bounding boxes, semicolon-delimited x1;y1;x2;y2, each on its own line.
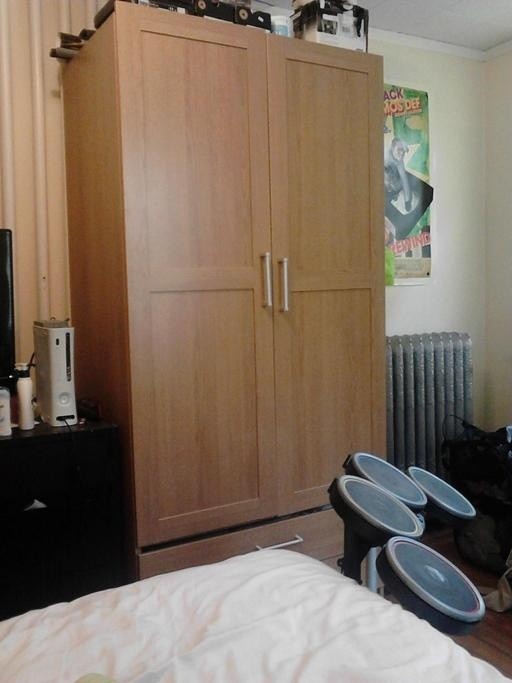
288;0;368;54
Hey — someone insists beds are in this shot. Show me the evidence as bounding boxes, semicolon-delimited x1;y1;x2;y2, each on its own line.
0;548;512;683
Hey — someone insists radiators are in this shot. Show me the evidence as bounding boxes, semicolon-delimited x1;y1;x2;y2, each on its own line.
385;332;474;478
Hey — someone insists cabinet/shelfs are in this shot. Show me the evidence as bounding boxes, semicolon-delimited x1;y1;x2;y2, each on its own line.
62;0;388;598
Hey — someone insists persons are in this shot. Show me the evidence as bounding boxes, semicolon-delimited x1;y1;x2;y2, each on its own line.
384;136;412;216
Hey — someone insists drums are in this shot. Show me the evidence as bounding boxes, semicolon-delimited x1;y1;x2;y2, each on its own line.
343;452;427;510
406;466;477;520
375;536;485;635
327;474;423;547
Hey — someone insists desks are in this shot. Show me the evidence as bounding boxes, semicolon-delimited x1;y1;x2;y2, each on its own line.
0;403;122;597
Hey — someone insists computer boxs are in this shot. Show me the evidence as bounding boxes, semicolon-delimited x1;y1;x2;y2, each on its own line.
33;325;78;428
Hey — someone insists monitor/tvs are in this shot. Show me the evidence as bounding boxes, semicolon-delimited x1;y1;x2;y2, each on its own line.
0;229;16;385
